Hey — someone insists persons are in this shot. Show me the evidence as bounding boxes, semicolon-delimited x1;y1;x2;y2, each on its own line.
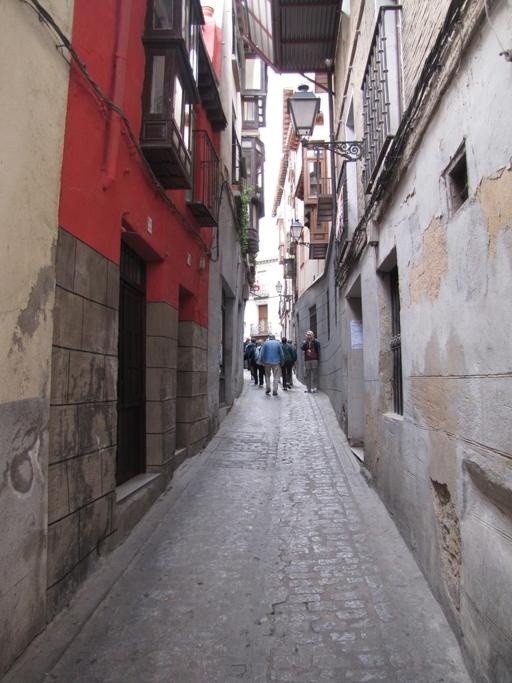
244;332;297;395
300;329;320;393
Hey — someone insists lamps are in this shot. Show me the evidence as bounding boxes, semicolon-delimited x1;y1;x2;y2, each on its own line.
275;281;293;298
290;218;313;252
287;84;364;163
